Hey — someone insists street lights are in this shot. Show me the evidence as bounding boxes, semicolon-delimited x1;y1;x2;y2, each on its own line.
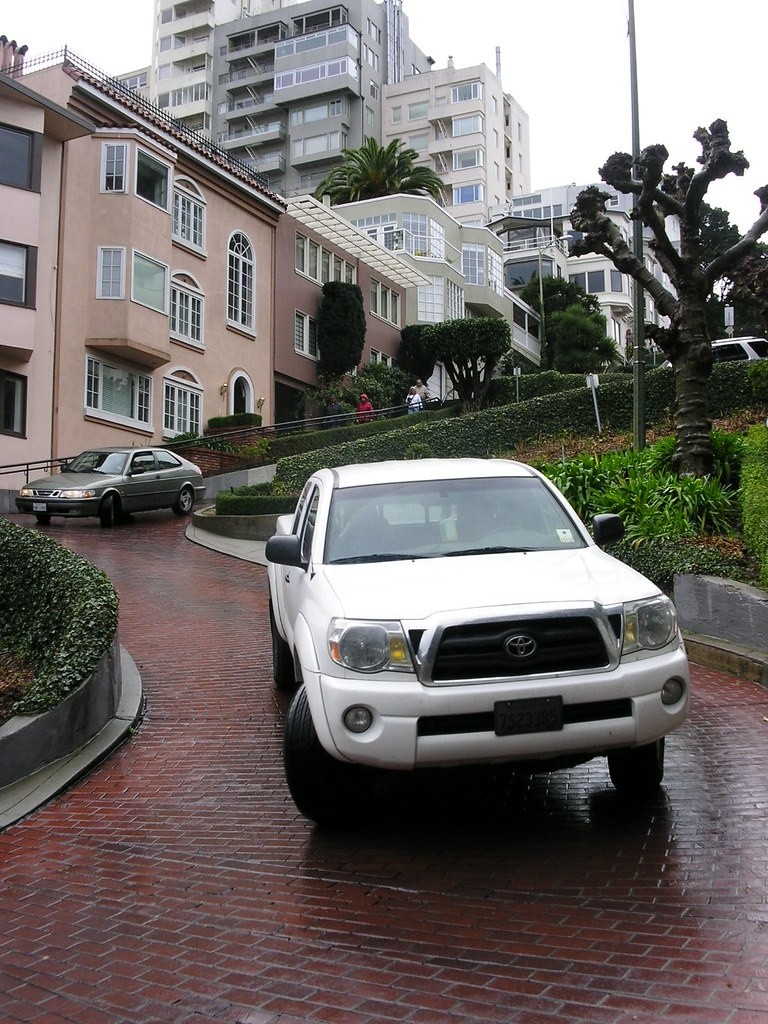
539;235;574;335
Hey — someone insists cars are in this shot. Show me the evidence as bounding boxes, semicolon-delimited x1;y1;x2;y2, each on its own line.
16;448;209;528
263;460;691;835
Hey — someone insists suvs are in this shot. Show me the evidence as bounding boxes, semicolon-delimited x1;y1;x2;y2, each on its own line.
652;335;768;373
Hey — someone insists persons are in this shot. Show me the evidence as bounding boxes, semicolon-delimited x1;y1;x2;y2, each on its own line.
356;393;374;424
414;379;430;411
325;395;347;429
129;453;144;473
405;386;423;414
